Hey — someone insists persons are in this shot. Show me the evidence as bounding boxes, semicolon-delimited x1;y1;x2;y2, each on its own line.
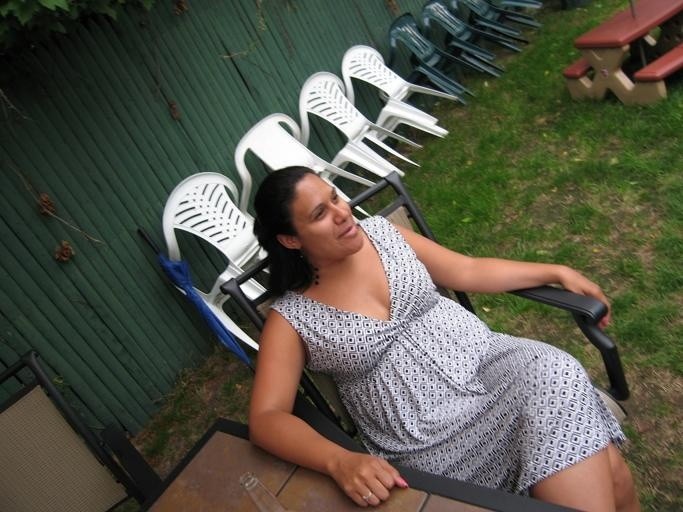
246;164;643;512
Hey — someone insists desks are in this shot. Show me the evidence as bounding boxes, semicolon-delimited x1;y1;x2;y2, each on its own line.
138;417;584;511
573;0;683;105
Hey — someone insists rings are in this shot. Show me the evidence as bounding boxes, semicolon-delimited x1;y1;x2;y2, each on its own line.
361;492;372;501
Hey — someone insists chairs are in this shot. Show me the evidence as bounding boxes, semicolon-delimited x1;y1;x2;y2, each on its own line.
218;169;628;436
166;0;546;372
0;349;162;511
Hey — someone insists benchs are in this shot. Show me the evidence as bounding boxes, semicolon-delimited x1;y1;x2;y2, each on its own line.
561;40;683;107
